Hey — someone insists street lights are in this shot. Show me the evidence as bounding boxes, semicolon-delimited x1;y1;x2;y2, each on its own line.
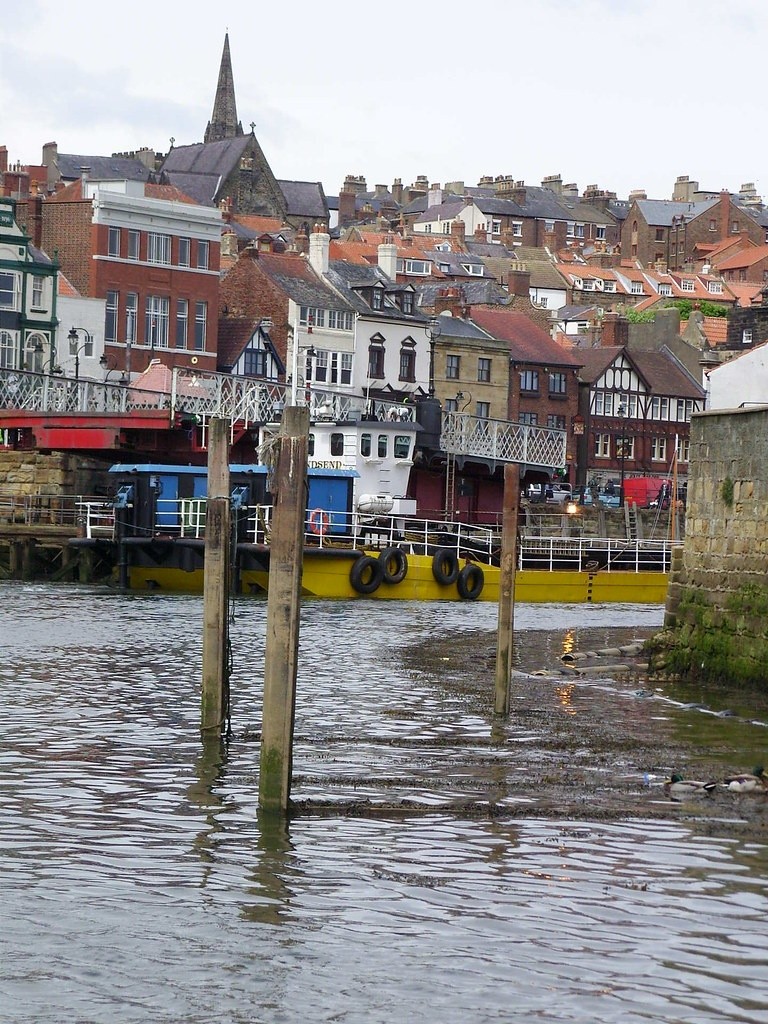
67;325;89;379
98;352;119;412
616;402;633;507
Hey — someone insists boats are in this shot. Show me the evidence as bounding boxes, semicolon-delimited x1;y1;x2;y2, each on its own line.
67;495;685;605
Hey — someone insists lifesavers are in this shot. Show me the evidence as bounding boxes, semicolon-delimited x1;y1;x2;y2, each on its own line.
349;555;384;594
310;508;329;534
457;564;484;600
431;548;460;586
377;547;409;585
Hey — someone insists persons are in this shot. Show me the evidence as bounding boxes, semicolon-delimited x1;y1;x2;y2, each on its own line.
661;480;669;490
605;478;615;494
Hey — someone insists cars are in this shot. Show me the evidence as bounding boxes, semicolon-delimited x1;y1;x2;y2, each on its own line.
526;482;620;508
